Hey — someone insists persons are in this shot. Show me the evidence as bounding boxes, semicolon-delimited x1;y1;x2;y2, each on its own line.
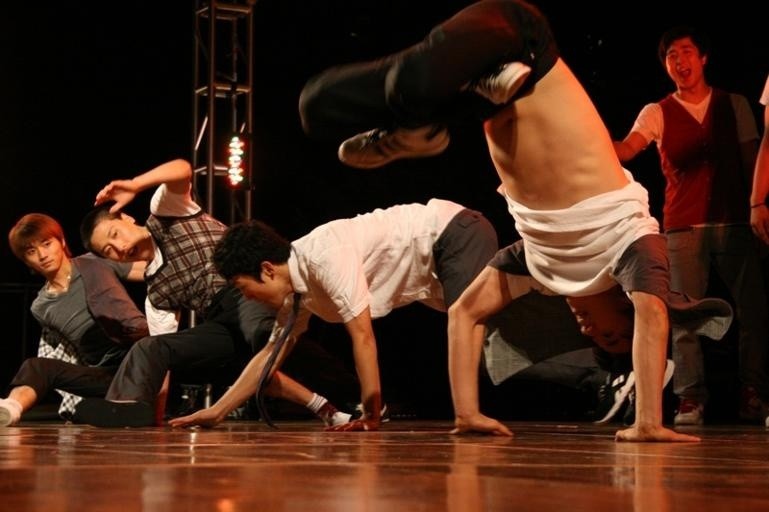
1;212;354;429
163;196;676;429
73;159;276;429
296;2;705;443
609;21;762;427
748;73;769;247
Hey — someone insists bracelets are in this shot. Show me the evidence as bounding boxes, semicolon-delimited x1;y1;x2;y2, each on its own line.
749;201;767;210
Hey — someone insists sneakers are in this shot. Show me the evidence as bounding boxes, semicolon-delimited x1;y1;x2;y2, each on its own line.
338;125;450;168
625;359;675;425
473;62;532;105
323;412;351;427
676;398;703;425
591;370;635;424
75;398;154;428
0;399;20;427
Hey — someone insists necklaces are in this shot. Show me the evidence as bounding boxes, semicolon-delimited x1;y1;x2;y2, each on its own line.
66;274;71;284
